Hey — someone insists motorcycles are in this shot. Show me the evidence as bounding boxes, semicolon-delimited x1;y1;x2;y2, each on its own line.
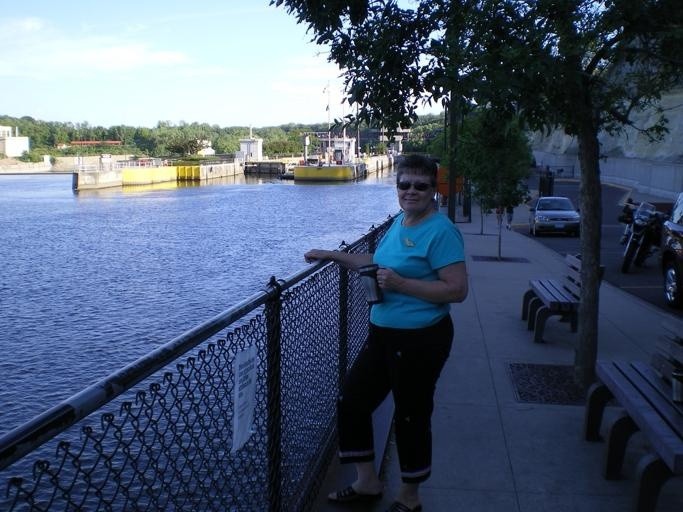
618;202;669;273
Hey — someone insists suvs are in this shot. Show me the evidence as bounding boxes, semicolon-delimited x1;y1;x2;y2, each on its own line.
658;192;682;307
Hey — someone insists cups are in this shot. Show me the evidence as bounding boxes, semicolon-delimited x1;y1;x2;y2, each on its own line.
358;264;390;306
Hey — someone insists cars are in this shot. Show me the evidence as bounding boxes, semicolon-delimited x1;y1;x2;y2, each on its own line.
528;197;581;238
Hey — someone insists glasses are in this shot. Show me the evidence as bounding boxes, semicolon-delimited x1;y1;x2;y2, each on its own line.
397;180;430;191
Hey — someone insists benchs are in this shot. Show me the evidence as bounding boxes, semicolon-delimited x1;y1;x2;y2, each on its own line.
584;312;683;512
522;252;605;343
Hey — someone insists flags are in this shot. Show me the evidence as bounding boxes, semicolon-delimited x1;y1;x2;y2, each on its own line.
325;105;329;112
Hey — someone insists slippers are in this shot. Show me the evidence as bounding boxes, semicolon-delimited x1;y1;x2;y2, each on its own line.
385;499;424;512
329;486;382;501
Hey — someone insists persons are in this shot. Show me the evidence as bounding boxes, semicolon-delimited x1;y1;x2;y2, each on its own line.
301;153;468;511
